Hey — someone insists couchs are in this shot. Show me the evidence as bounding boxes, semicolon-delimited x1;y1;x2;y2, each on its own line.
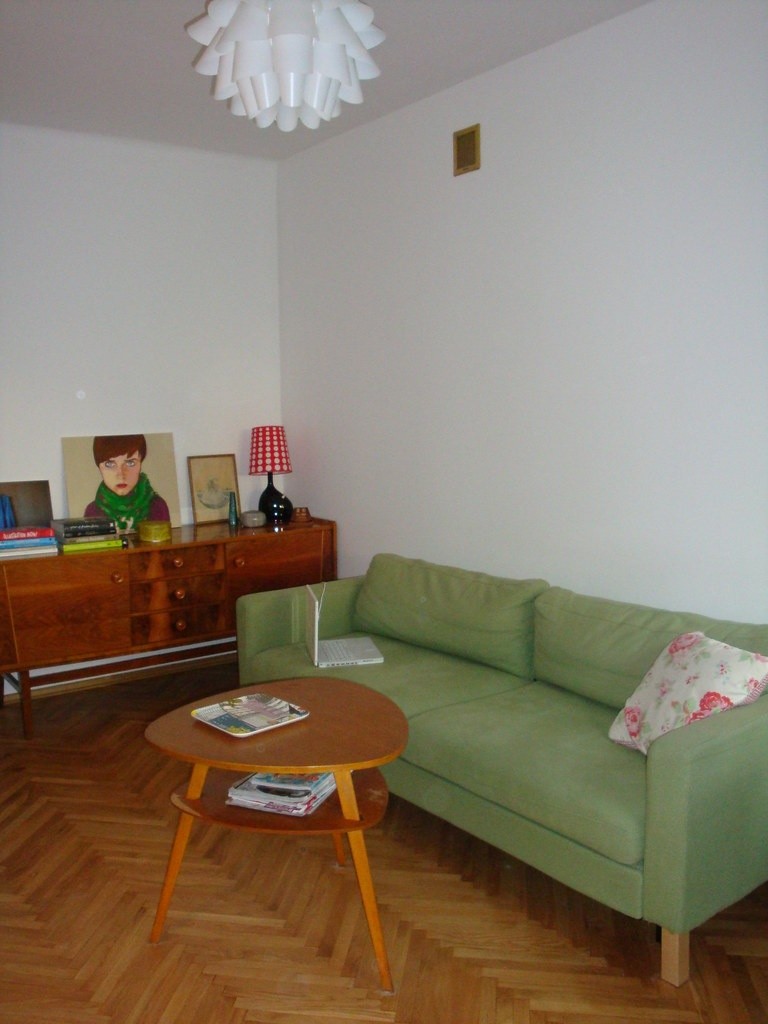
235;576;768;984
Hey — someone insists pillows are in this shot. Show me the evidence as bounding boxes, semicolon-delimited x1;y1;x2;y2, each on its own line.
355;552;549;679
531;588;768;710
603;631;768;754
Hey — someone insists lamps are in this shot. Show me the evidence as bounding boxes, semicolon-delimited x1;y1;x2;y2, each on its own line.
186;0;385;132
248;425;293;522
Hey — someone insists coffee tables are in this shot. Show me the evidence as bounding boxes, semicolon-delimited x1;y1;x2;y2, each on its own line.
146;675;410;991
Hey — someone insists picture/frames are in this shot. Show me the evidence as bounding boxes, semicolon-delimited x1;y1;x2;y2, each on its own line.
187;453;242;527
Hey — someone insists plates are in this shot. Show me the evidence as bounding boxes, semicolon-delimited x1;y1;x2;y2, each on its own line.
192;693;310;738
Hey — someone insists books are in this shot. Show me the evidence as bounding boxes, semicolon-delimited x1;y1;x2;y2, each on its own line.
225;773;337;818
50;517;123;552
0;526;60;559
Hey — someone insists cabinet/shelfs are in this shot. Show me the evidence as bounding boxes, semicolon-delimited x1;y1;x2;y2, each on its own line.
0;517;338;739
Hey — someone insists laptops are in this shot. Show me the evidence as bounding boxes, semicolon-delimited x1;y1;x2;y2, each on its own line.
305;585;384;667
